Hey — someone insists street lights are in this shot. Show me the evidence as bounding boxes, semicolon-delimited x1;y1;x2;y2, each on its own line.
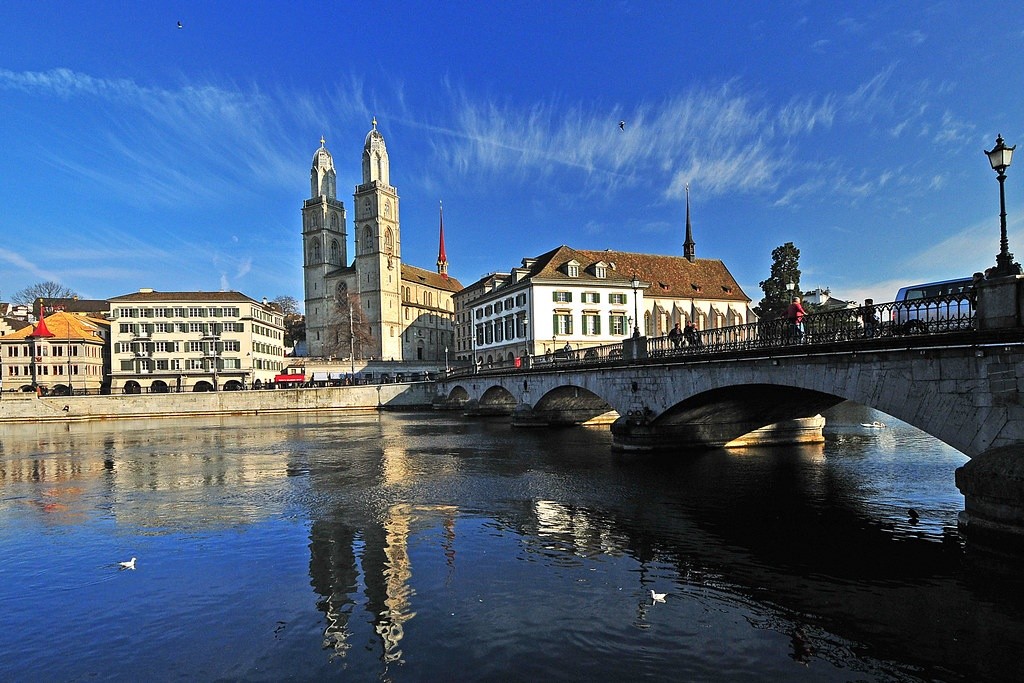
630;273;641;338
445;346;449;373
523;316;528;358
786;277;795;306
552;335;556;353
473;336;476;367
350;292;361;387
982;133;1023;277
628;316;633;339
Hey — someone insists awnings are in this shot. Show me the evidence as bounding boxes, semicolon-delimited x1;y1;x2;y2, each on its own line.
275;375;305;382
330;372;344;379
313;372;328;381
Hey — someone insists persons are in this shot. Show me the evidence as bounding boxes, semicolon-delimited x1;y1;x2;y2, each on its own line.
424;371;429;381
564;342;572;360
668;322;700;352
346;378;355;386
544;348;552;361
859;299;876;337
779;297;807;345
37;386;49;396
968;269;992;327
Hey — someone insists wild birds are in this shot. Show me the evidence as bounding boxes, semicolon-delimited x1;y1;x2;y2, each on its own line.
176;20;183;29
617;119;627;131
649;589;669;599
646;598;667;607
118;558;138;567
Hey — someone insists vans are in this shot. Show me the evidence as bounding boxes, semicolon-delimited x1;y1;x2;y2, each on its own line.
893;276;975;335
